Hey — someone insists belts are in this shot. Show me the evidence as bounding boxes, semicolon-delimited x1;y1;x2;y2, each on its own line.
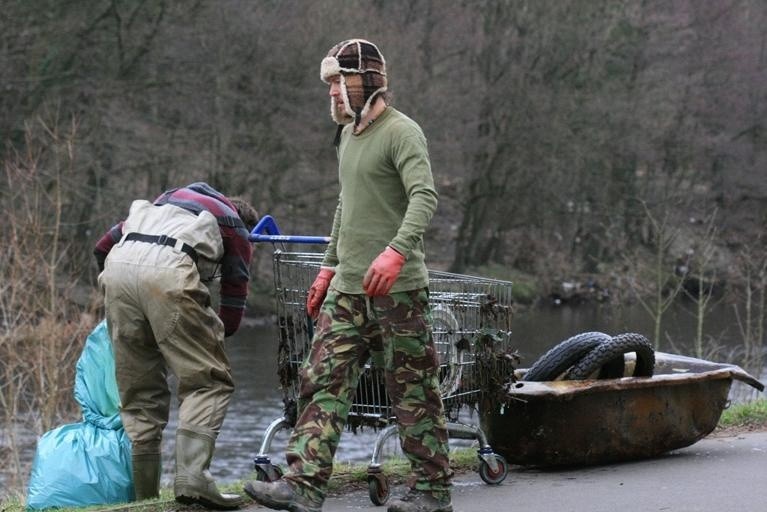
124;231;199;265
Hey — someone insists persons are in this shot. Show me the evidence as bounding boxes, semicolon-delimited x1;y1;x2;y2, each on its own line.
243;37;456;512
92;181;243;507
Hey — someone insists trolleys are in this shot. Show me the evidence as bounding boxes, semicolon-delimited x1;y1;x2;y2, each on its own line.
248;213;514;507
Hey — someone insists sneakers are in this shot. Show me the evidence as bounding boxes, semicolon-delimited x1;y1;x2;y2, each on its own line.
386;487;455;512
244;478;323;511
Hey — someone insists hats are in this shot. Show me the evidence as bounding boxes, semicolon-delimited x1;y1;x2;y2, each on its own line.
319;37;389;125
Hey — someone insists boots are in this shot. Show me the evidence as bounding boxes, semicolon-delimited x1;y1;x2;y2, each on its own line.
130;447;163;504
173;420;242;509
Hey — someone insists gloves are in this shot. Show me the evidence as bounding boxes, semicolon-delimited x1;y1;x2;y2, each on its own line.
362;245;408;297
306;269;335;319
